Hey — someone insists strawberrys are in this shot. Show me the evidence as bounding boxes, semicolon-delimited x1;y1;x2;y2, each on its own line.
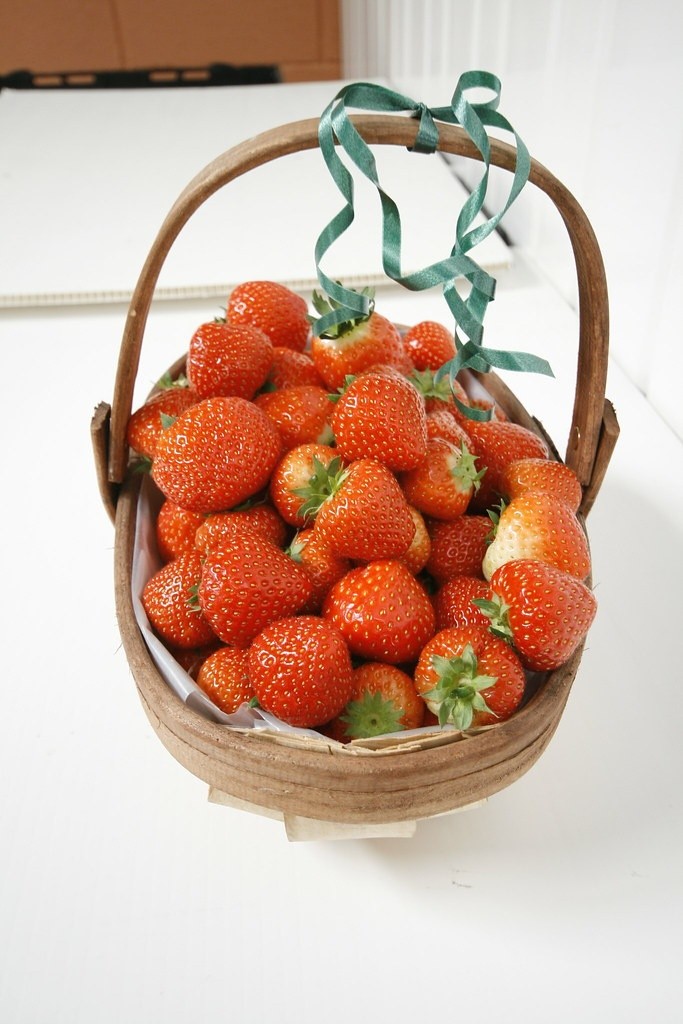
130;283;598;744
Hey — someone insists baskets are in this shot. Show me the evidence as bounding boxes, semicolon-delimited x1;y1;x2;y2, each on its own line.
87;114;622;825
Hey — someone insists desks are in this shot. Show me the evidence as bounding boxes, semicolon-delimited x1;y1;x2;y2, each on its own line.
0;78;681;1022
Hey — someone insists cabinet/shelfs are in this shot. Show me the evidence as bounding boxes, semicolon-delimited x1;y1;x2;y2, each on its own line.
1;1;344;84
